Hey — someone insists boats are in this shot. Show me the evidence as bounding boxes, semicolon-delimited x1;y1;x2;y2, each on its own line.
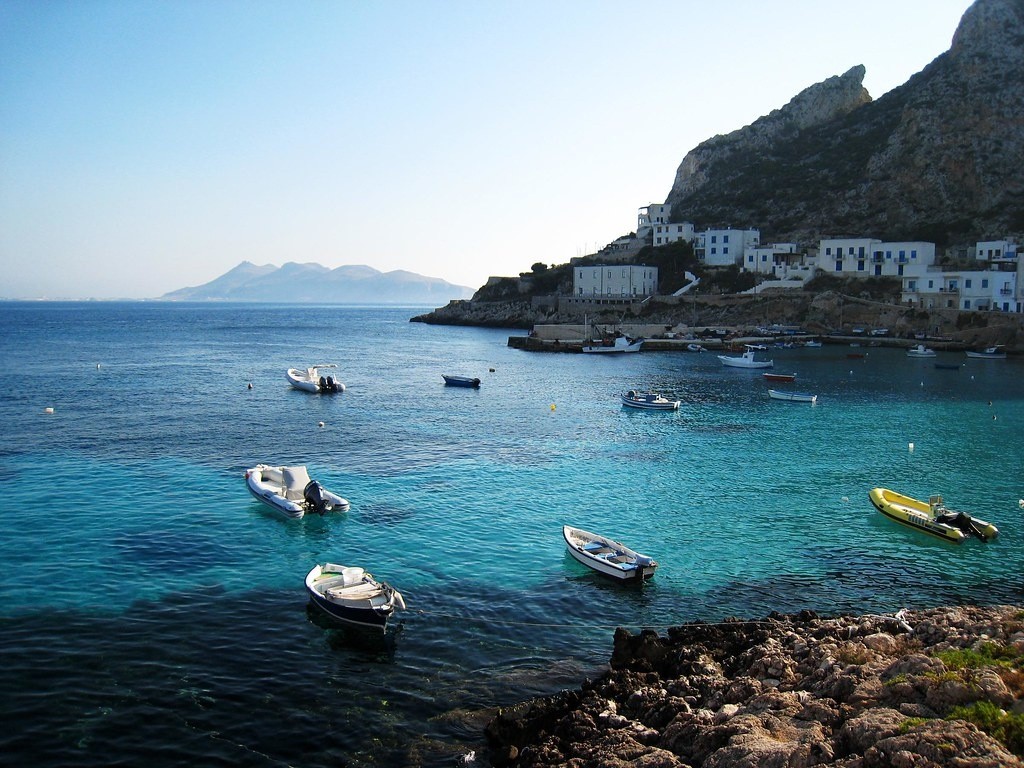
866;487;999;545
851;343;861;348
688;344;707;353
936;363;961;370
782;340;820;348
304;562;406;635
965;351;1008;359
561;525;658;589
767;389;818;402
716;344;776;369
763;372;794;383
285;364;346;395
580;325;643;354
906;343;938;358
620;389;682;411
443;372;482;388
244;462;350;519
